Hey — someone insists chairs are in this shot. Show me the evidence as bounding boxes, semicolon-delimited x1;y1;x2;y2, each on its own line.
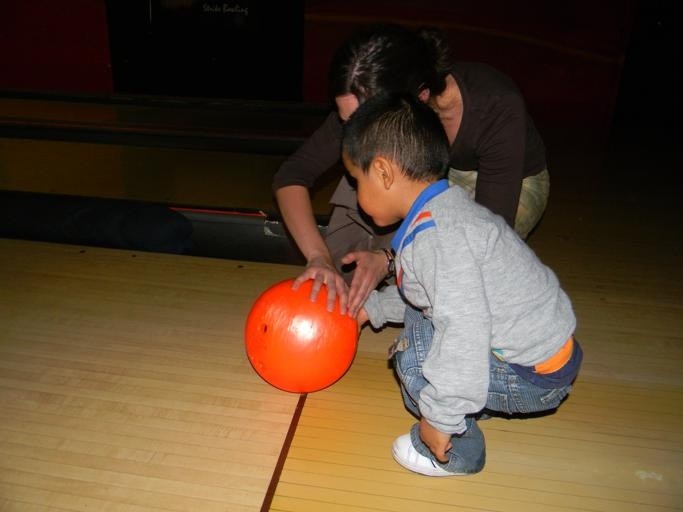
392;434;468;477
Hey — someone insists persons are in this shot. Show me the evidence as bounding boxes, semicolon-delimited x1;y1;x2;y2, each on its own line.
270;14;554;319
338;89;583;481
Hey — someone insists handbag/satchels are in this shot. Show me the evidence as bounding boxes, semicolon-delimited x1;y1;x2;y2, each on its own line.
378;247;398;284
